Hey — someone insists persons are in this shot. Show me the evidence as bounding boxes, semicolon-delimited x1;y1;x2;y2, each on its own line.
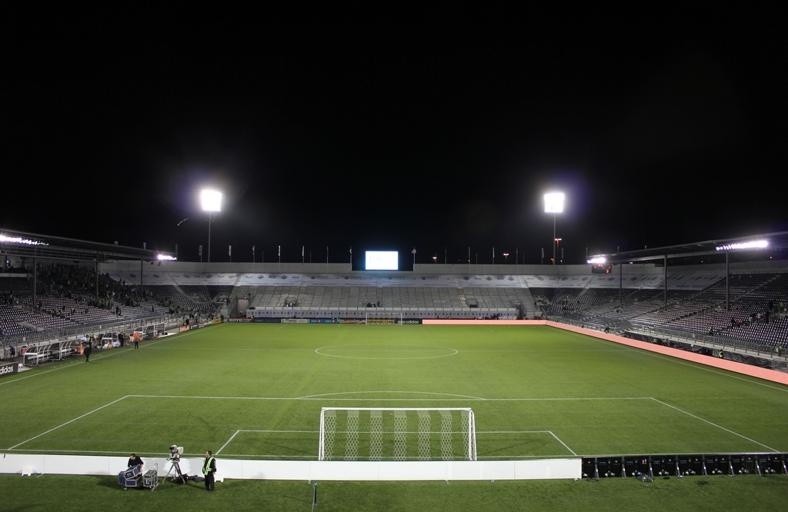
202;451;217;490
0;272;224;362
603;298;787;357
128;453;143;469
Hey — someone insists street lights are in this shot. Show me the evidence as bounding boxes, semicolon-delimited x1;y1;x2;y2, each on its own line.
543;192;565;265
199;189;222;263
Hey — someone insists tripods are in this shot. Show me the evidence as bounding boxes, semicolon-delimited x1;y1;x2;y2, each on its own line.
161;460;187;486
